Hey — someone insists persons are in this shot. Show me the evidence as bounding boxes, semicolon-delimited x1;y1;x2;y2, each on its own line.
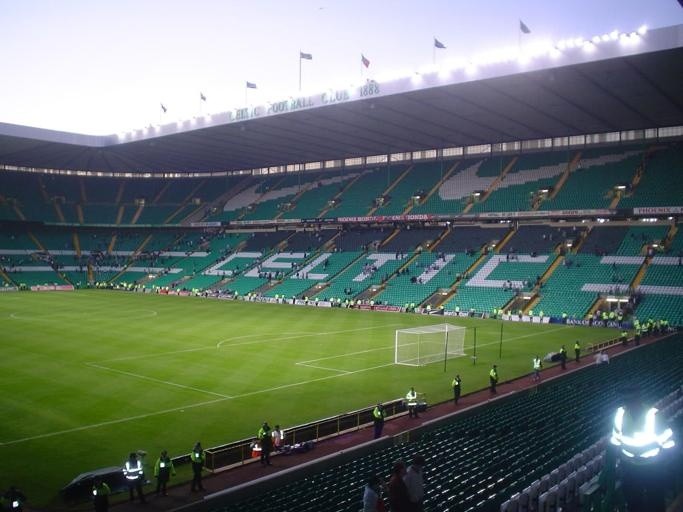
559;344;569;370
621;330;627;347
0;485;27;511
634;329;640;345
362;476;388;511
404;386;419;418
271;424;281;452
574;339;581;363
121;452;150;505
596;350;603;367
387;461;416;511
451;374;461;403
257;420;268;440
624;330;627;341
153;449;177;497
605;381;678;511
91;474;113;512
531;355;542;382
259;425;272;465
372;401;386;438
400;453;428;510
601;350;609;365
190;440;207;492
489;364;499;394
1;222;682;336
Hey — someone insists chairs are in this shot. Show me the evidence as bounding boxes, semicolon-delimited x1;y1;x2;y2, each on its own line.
166;332;682;512
0;138;683;331
